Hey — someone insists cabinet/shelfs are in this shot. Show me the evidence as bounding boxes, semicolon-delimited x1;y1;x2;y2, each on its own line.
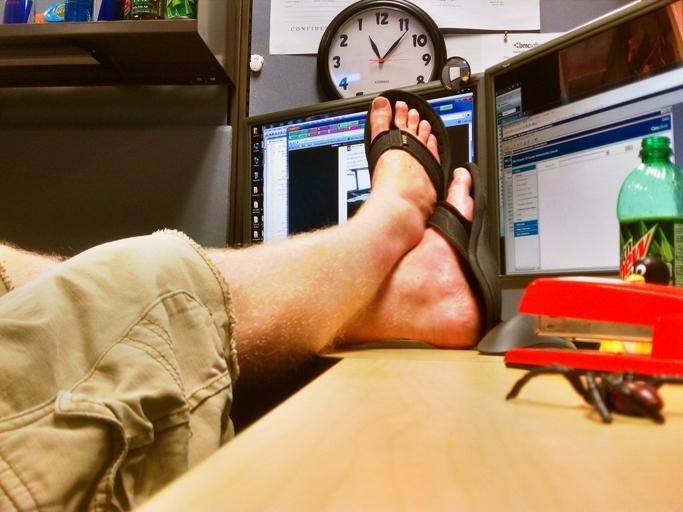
0;2;240;94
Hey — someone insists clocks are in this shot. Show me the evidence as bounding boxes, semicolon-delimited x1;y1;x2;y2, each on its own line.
317;0;449;102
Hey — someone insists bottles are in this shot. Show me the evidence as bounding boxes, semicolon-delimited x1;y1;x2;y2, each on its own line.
617;135;683;286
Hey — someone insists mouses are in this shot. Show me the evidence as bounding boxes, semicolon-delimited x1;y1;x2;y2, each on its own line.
477;313;577;355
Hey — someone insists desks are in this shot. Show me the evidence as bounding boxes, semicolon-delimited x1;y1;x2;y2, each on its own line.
135;343;681;510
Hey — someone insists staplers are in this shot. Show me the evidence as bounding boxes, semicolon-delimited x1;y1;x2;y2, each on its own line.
504;278;683;383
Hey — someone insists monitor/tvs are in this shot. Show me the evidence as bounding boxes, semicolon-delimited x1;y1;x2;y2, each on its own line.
484;0;683;289
241;73;489;249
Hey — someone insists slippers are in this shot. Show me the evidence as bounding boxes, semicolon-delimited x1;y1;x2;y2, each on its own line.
363;88;452;203
424;160;504;340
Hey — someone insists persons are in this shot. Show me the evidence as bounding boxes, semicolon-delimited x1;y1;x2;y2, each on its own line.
0;91;502;512
